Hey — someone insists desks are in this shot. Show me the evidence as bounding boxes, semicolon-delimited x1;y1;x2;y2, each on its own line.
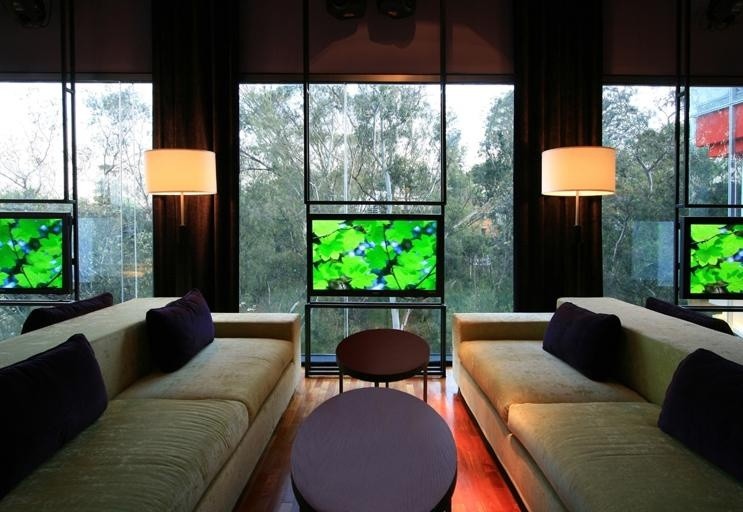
289;386;460;512
335;327;430;403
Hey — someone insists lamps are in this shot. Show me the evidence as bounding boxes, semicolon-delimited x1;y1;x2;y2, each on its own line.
139;147;218;233
540;145;618;242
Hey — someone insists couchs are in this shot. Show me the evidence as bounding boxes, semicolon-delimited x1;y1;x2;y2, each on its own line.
450;294;742;512
0;295;302;511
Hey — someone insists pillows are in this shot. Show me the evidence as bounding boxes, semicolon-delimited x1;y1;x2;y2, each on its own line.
21;291;113;335
656;345;743;484
541;302;625;384
641;294;735;336
0;331;108;499
145;286;217;375
